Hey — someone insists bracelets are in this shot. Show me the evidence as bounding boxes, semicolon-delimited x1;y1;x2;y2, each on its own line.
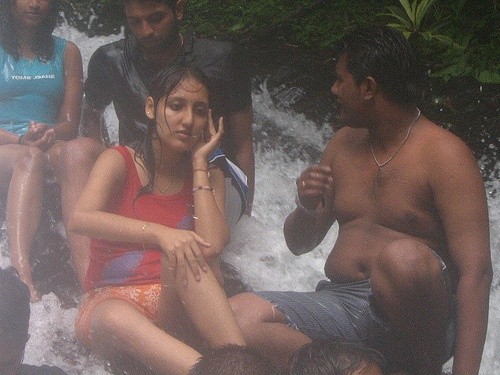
295;194;328;218
140;221;149;240
17;135;23;145
190;168;212;178
190;186;214;193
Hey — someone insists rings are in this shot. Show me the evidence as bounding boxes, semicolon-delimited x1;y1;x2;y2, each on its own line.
301;181;306;190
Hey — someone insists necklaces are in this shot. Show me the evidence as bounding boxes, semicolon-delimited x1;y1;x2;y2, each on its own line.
366;107;422;166
154;174;178;194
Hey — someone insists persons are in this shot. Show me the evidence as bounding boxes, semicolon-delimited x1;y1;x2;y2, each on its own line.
226;24;493;375
0;0;83;304
284;336;388;375
60;0;255;289
188;343;282;375
65;65;248;375
0;267;68;375
